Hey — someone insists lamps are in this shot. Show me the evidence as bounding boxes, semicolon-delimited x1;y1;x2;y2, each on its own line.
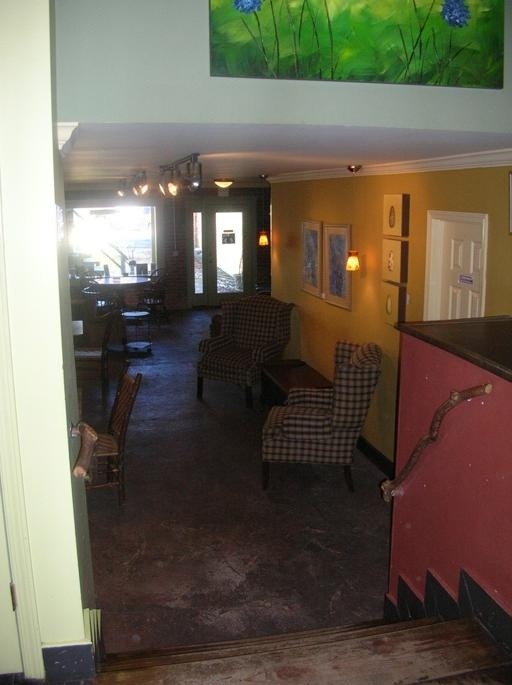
213;178;234;188
116;170;149;197
258;230;270;246
158;151;203;200
345;249;362;272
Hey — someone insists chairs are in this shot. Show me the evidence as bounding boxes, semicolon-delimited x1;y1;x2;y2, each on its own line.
71;310;129;409
261;341;383;489
121;309;152;355
76;362;143;500
194;293;294;410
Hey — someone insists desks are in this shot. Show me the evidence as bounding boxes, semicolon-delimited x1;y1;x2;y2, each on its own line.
89;275;151;351
262;358;352;405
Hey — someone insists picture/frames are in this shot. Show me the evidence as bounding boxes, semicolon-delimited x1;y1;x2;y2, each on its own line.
299;217;322;296
322;223;352;312
